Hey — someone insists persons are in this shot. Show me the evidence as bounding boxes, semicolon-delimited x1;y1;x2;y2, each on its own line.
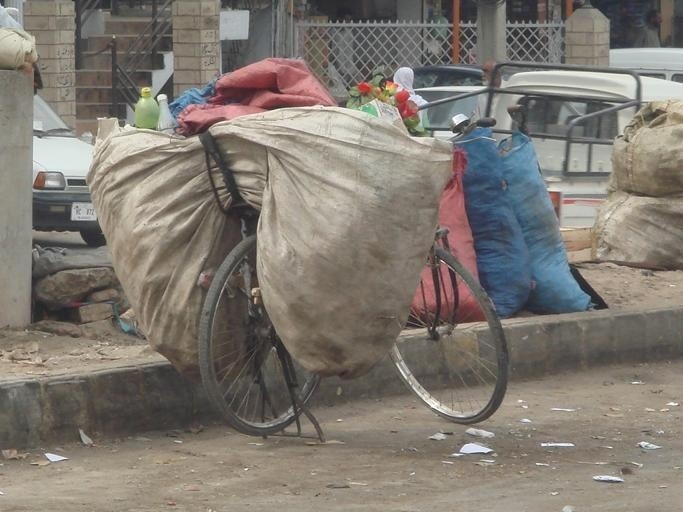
391;68;429;128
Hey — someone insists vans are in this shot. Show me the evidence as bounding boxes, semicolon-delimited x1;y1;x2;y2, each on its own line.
609;47;683;82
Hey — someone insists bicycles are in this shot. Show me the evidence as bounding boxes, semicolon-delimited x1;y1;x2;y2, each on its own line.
197;112;509;447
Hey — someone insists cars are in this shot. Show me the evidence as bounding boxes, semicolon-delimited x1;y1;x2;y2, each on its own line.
382;65;490;139
31;88;105;246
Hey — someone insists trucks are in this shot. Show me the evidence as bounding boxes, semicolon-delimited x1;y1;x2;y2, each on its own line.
399;60;683;231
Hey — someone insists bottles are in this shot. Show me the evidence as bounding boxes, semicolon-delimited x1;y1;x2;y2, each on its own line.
133;87;180;134
